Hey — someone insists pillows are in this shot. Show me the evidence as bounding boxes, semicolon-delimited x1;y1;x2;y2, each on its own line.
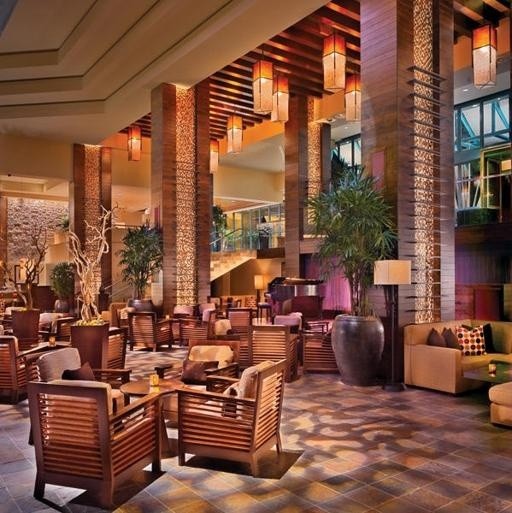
112;396;125;436
428;324;497;356
222;381;242;421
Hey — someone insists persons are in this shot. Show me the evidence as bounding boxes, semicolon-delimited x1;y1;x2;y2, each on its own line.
222;213;230;251
256;215;273;251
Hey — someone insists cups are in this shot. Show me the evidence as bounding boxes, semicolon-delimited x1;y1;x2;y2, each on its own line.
48;335;56;346
149;373;160;386
489;363;497;377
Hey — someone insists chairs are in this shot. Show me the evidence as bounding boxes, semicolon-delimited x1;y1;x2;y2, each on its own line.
25;379;166;510
152;339;241;425
174;358;287;477
21;345;125;447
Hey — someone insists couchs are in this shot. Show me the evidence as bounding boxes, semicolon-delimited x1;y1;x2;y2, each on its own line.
488;382;512;427
404;317;512;395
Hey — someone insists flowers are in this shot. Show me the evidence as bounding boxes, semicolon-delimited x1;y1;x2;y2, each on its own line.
0;195;72;313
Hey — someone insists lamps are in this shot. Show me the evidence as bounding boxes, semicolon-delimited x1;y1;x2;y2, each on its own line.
469;1;498;88
226;100;243;158
372;258;413;392
252;26;361;124
208;127;220;174
126;122;141;162
253;274;263;306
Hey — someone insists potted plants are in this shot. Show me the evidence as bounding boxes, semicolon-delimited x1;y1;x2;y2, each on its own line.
114;222;162;321
300;163;401;388
49;261;75;313
65;201;119;370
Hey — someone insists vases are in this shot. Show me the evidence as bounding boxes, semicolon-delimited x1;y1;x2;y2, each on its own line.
11;308;42;351
